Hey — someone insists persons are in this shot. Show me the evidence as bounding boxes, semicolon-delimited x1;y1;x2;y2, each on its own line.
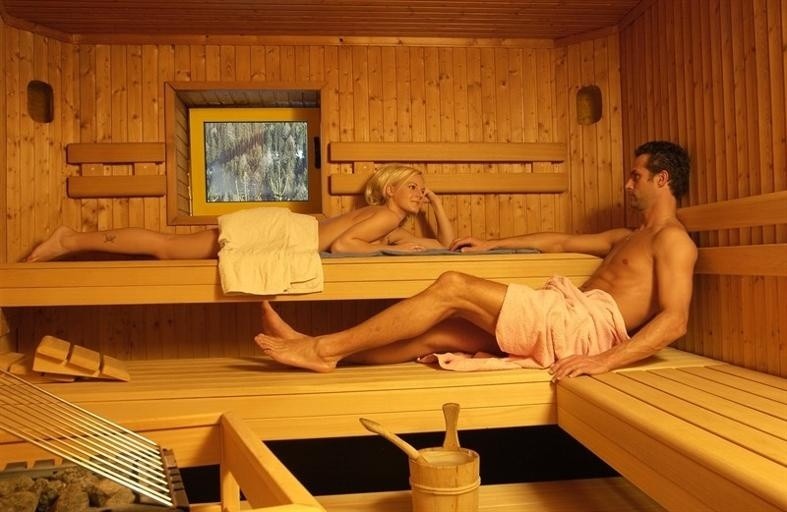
254;140;698;383
27;166;453;264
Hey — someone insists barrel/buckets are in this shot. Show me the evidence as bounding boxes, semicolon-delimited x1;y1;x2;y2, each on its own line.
409;447;481;512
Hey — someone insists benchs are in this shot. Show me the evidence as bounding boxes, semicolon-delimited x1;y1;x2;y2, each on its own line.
1;142;787;512
